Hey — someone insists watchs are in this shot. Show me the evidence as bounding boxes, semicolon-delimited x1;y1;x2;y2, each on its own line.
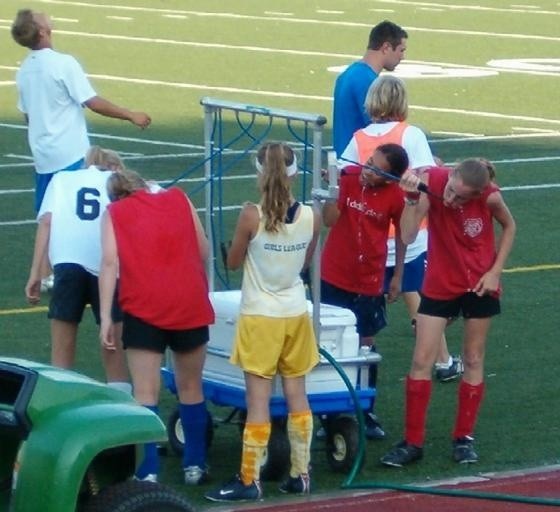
404;198;417;206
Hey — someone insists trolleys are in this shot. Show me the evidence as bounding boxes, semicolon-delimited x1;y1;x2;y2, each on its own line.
158;96;381;472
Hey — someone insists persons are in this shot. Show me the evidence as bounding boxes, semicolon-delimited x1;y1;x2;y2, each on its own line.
99;169;216;487
24;145;133;397
11;8;152;292
333;75;466;383
202;141;321;504
331;19;446;169
305;142;409;441
378;157;516;469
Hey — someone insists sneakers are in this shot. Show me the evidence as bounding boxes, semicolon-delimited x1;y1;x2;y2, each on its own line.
39;273;55;292
380;440;423;467
364;414;385;439
452;433;479;464
279;467;314;496
204;474;263;502
316;427;328;439
183;465;211;485
132;473;158;482
434;355;465;383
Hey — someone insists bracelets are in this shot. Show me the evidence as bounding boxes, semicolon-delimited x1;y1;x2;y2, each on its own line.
407;191;420;200
